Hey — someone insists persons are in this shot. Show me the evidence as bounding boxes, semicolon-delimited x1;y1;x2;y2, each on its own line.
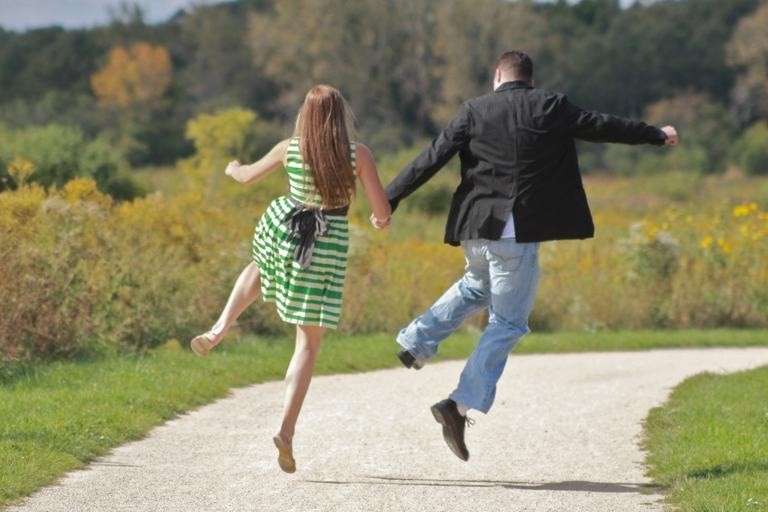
190;85;392;474
369;51;678;462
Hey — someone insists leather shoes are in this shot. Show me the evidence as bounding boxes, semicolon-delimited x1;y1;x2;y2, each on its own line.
397;349;420;371
431;398;475;461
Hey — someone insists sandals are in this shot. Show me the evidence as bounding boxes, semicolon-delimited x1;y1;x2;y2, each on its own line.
273;435;296;473
190;331;223;356
